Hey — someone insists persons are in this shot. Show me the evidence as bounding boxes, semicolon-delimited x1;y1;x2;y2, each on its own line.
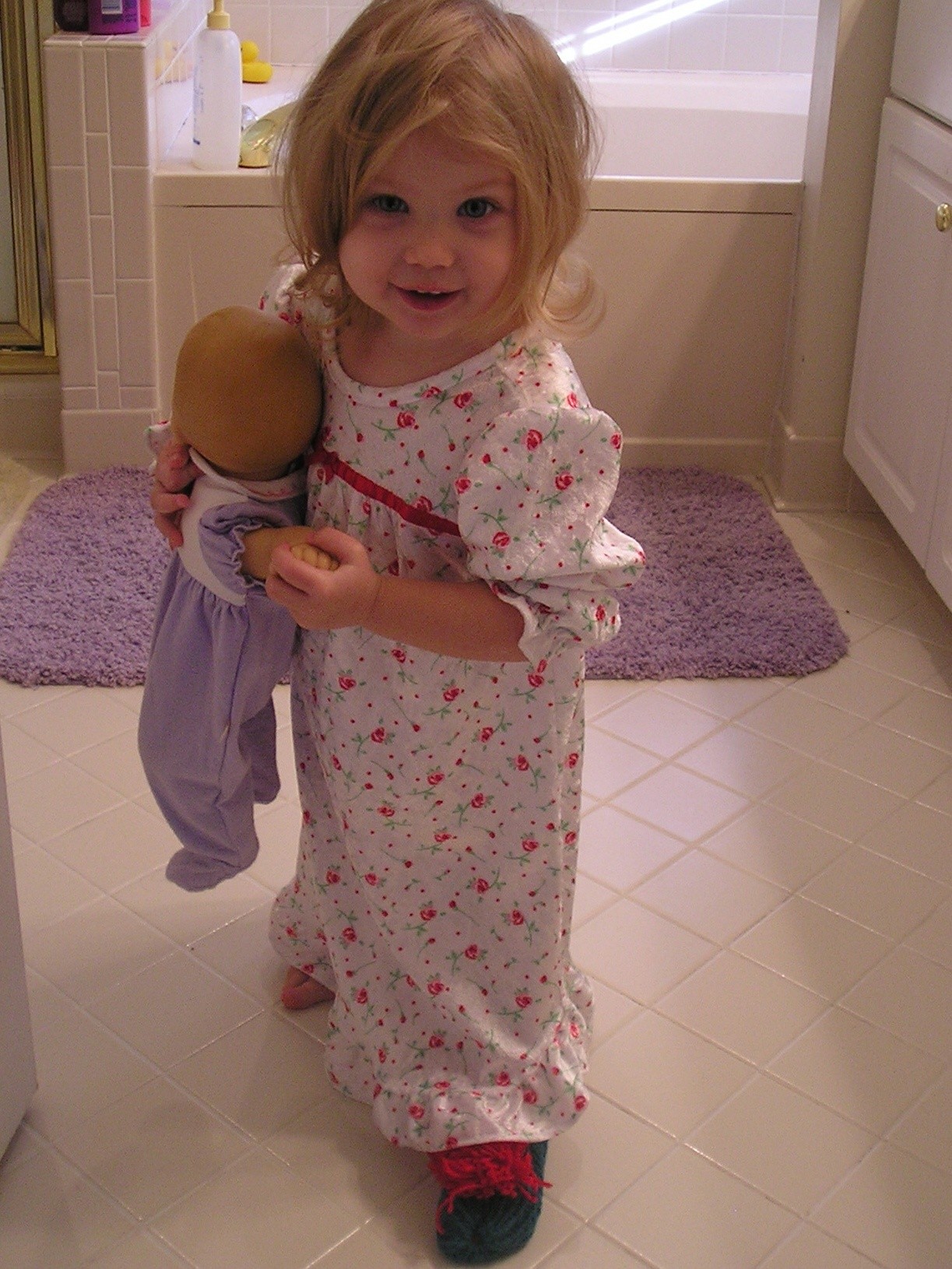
145;0;646;1260
134;308;342;893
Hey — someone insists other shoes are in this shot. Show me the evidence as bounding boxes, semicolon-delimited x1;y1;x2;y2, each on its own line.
427;1134;549;1266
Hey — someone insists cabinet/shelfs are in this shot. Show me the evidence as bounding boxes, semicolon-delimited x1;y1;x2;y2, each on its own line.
843;2;951;613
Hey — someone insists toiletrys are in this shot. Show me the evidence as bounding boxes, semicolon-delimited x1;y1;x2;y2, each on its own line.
55;1;153;36
190;0;246;175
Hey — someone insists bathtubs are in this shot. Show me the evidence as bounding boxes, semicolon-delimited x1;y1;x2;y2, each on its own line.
153;68;814;441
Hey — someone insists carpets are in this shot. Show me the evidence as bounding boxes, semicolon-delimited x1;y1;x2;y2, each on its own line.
0;472;852;690
